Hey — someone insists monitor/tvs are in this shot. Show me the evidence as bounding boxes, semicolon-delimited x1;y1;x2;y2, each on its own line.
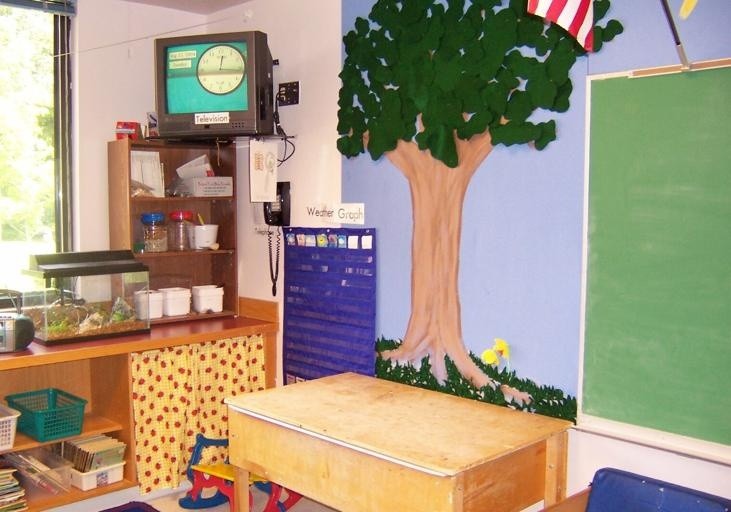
155;30;274;138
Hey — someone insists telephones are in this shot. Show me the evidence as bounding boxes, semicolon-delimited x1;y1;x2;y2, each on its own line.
263;182;290;226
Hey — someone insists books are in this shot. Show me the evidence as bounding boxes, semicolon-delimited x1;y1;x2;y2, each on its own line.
1;434;127;512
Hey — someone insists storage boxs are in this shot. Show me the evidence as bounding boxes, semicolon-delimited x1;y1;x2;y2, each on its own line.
21;251;150;343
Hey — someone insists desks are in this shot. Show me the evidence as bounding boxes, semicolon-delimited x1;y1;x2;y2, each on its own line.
224;371;575;512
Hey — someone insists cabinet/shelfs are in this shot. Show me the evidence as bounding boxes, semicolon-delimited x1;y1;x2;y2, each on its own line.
0;321;137;512
108;138;238;325
127;329;277;485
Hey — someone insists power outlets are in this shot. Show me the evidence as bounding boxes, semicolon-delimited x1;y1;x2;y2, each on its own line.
278;81;299;107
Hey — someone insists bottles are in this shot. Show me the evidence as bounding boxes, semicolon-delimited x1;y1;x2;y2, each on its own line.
139;209;196;255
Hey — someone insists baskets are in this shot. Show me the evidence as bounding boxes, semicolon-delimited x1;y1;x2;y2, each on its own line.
3;403;23;451
6;388;88;444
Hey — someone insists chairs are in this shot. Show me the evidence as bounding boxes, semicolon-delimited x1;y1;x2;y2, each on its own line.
537;467;731;512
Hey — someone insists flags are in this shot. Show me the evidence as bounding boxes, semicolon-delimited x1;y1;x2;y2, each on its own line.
526;0;594;52
